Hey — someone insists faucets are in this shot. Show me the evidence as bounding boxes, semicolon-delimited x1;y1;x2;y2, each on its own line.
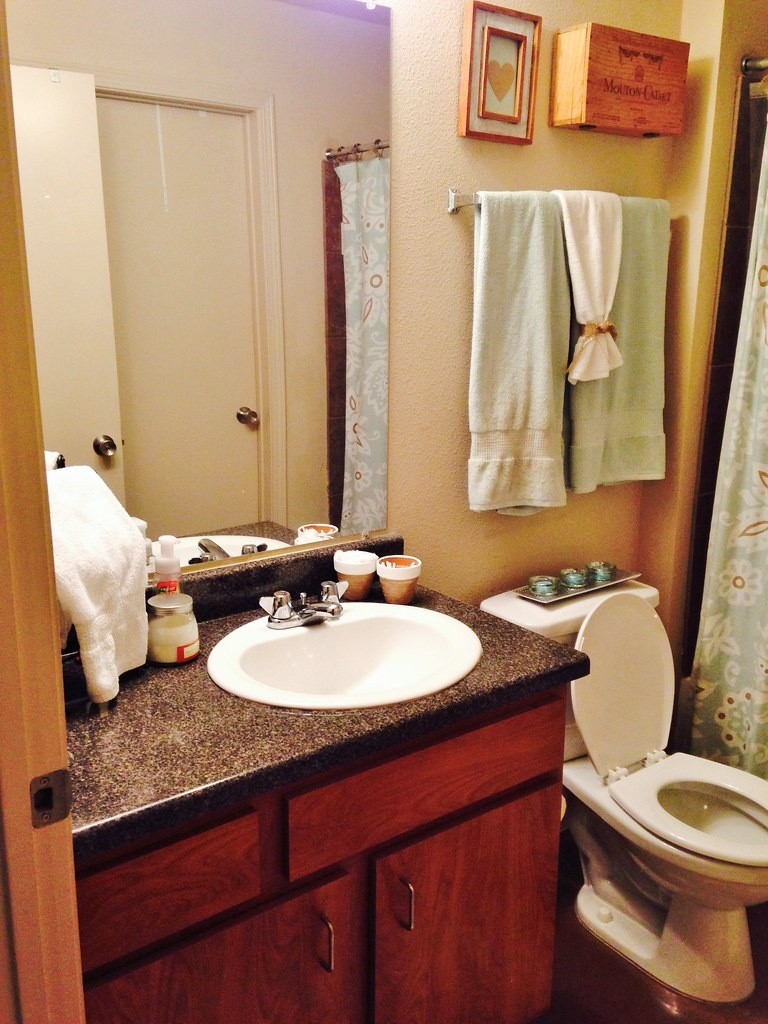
298;592;343;625
198;538;230;562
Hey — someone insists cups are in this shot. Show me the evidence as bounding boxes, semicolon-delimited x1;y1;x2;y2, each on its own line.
298;524;339;539
377;555;421;604
295;536;333;546
334;550;378;601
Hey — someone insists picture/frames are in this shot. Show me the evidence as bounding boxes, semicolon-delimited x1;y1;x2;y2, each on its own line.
457;0;545;146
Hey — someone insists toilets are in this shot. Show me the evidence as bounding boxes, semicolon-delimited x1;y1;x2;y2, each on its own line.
479;576;767;1001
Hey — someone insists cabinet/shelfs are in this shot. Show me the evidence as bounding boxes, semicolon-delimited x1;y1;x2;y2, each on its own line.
73;681;567;1024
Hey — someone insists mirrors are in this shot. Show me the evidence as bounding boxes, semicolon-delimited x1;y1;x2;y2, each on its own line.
5;0;393;576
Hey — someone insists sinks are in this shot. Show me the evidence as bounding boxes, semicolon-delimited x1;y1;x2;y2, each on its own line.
205;601;483;710
140;534;292;564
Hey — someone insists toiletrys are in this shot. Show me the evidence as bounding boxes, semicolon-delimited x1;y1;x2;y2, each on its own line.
146;535;200;665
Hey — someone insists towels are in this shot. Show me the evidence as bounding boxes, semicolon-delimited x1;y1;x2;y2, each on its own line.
468;190;671;516
45;465;150;704
47;449;60;469
